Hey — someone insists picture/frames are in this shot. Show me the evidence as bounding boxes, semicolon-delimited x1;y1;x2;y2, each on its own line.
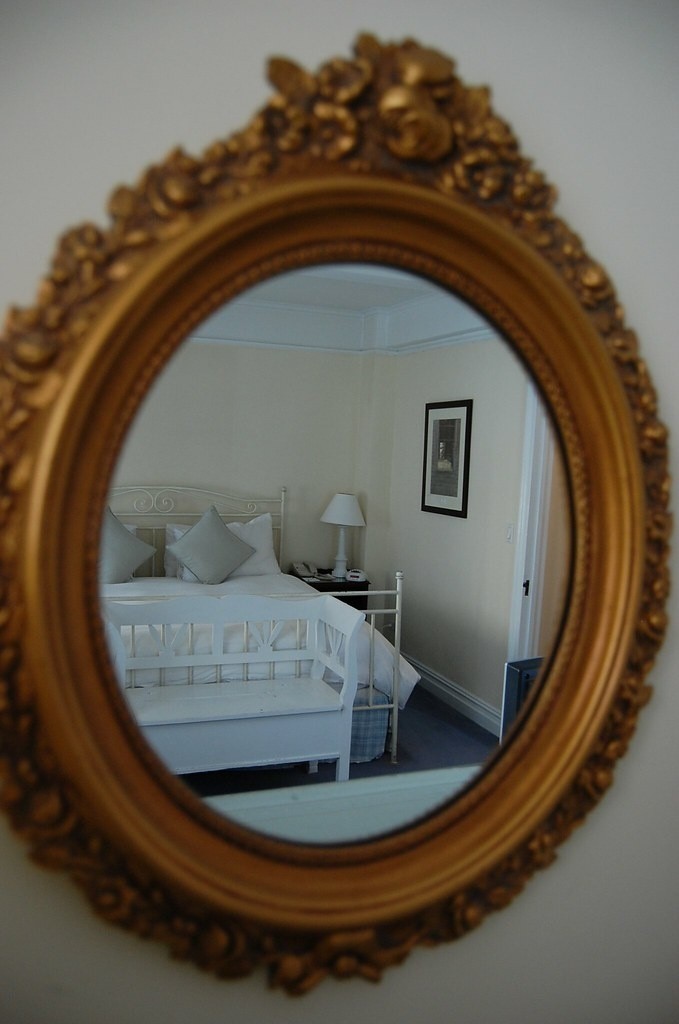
420;399;474;519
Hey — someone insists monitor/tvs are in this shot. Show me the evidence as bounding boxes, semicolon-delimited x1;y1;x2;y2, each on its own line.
500;657;544;746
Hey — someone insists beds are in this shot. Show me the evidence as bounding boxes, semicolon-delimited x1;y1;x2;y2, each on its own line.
99;484;422;770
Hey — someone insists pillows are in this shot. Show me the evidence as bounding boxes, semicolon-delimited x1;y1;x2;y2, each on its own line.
165;521;245;579
122;524;138;585
95;504;158;586
164;505;256;585
175;512;281;582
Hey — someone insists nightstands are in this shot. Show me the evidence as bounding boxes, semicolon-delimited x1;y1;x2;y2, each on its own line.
292;568;372;622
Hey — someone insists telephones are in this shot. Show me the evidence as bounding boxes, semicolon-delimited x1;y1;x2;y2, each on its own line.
292;561;318;577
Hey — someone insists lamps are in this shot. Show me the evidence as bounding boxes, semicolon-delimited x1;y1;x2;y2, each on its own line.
318;492;367;579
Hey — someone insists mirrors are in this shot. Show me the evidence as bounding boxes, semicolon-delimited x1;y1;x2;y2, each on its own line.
0;32;674;999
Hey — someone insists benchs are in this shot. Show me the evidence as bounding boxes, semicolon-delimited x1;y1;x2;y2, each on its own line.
101;594;367;783
